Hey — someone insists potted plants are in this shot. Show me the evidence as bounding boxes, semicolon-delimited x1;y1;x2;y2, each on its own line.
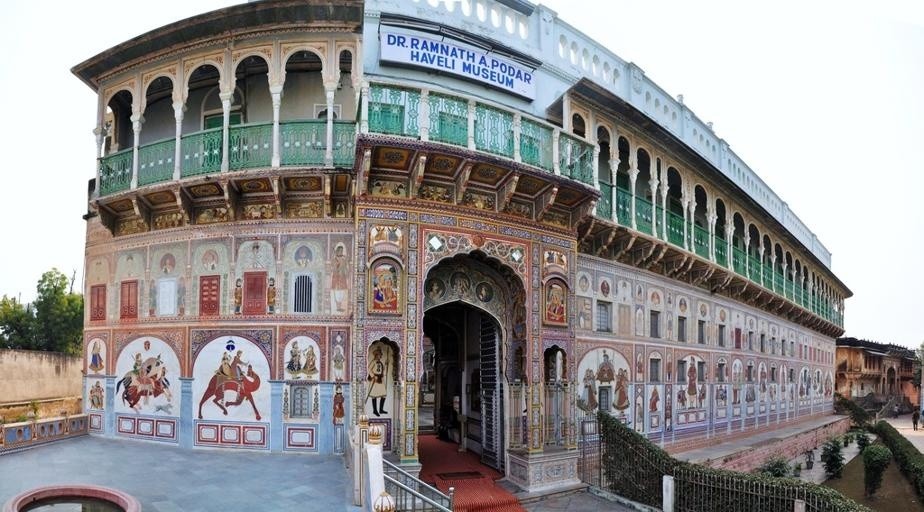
844;432;854;447
805;449;815;470
793;462;802;477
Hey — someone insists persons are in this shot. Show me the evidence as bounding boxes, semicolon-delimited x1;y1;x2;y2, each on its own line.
374;264;398;308
368;346;388;417
893;404;899;418
89;242;349;426
546;285;565;323
912;409;920;431
579;275;832;416
